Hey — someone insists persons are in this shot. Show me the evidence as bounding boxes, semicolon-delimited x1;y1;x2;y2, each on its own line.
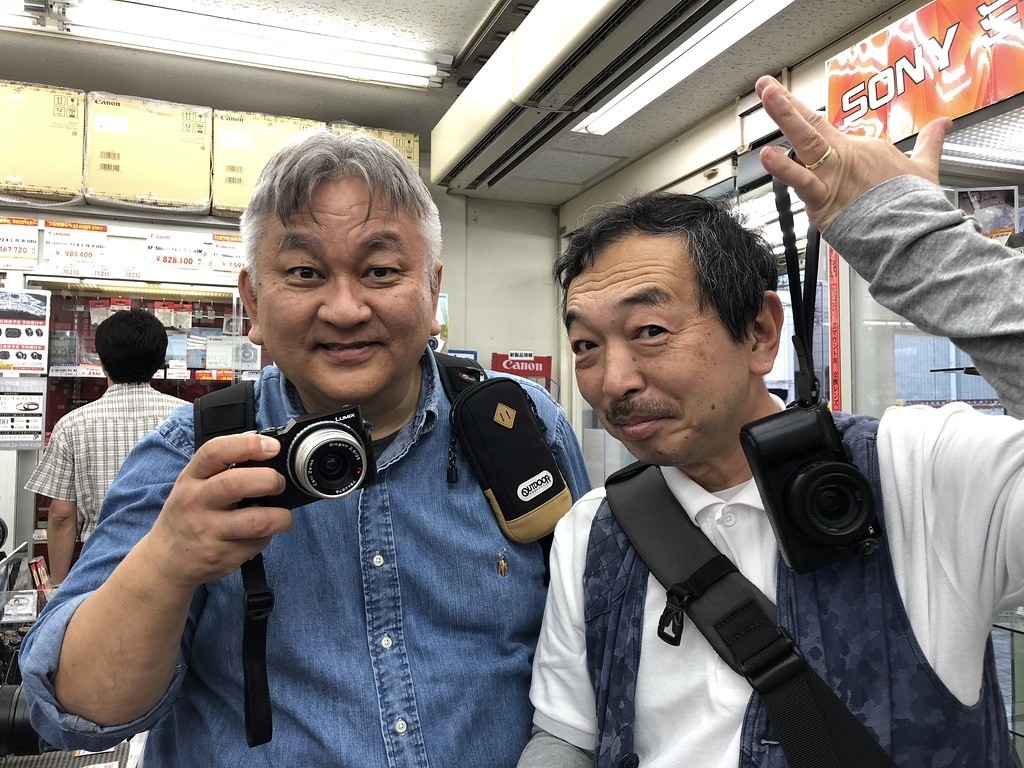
518;74;1023;768
24;307;193;588
17;126;593;768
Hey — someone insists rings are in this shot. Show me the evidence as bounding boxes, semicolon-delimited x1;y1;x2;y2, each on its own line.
807;145;832;171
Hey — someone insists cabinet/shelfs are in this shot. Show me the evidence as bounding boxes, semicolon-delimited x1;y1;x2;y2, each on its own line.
24;288;272;589
895;396;1023;749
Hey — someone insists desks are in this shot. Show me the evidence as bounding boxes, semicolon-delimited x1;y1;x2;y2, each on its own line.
0;589;58;684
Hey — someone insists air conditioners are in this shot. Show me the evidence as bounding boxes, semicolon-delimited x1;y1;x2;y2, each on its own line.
512;0;740;120
430;30;594;193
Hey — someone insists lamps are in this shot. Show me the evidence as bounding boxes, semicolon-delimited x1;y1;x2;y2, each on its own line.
0;0;454;92
570;0;797;136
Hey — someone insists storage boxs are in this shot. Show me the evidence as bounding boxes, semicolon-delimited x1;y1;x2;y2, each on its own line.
211;109;328;214
28;556;51;588
332;123;420;176
83;91;214;216
0;79;87;200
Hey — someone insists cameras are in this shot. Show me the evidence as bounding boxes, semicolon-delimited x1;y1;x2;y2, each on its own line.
233;342;258;363
740;399;881;576
239;403;378;510
